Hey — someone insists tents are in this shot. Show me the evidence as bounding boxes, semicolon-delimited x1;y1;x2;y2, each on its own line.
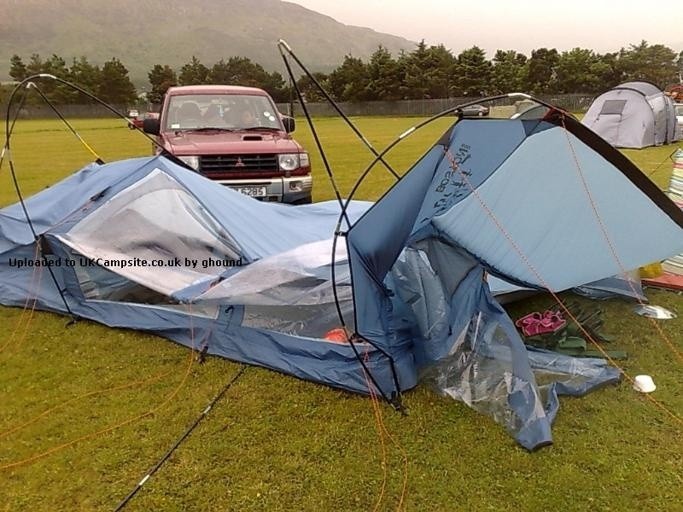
581;79;679;150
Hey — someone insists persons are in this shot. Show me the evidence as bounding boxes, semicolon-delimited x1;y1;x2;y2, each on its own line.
241;109;255;125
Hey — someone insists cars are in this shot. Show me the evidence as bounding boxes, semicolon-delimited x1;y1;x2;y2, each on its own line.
127;112;159;130
128;110;139;117
454;105;488;116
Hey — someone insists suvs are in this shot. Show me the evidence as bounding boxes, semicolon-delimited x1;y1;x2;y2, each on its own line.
142;84;313;205
